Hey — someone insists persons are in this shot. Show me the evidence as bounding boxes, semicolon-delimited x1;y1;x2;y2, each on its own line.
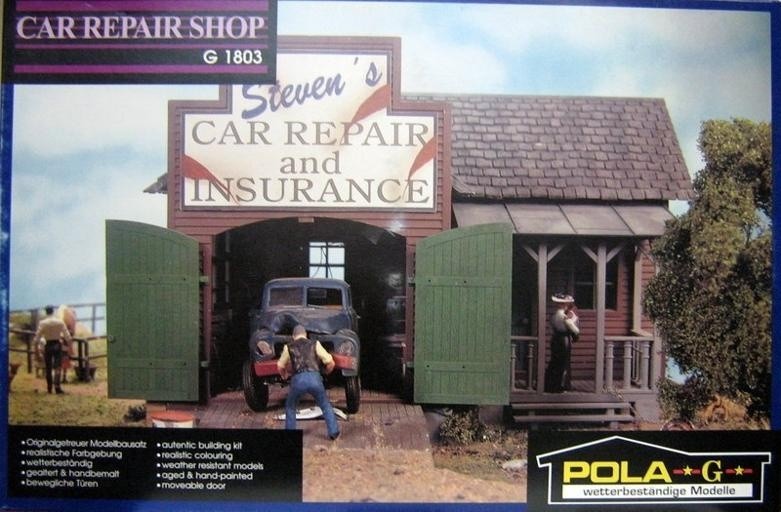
31;305;73;394
276;325;340;442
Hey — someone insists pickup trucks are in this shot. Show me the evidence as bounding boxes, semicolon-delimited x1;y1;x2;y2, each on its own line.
238;274;366;413
